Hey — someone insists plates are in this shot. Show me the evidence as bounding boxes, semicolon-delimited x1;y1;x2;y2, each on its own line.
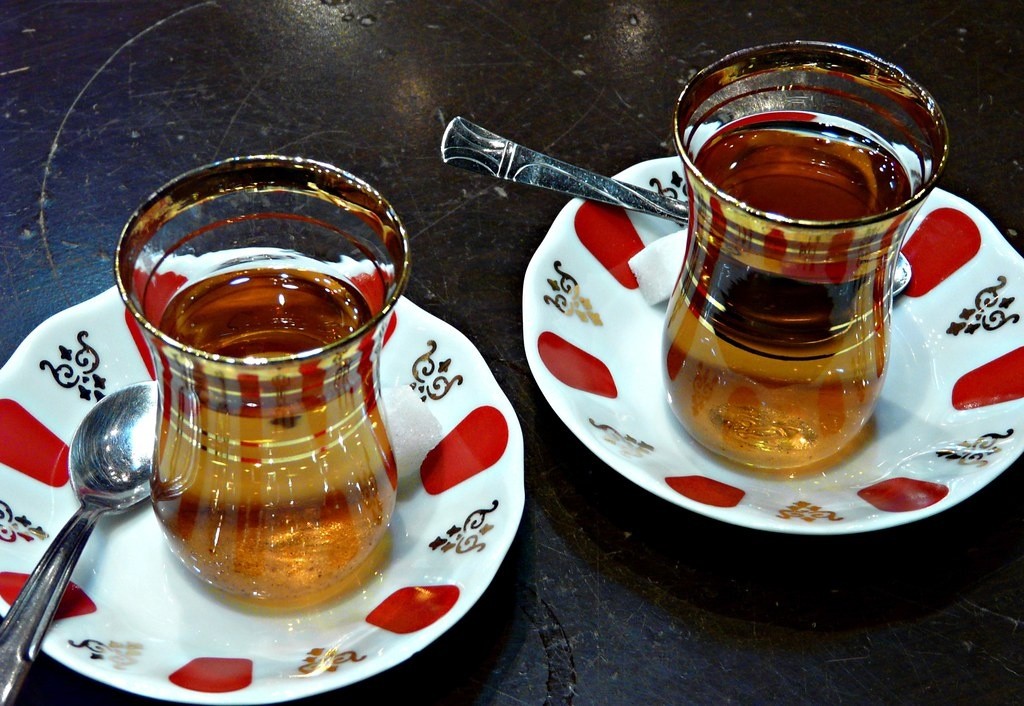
521;155;1023;537
0;283;528;706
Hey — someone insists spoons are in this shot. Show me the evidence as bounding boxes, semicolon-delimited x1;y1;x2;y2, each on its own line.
0;380;160;706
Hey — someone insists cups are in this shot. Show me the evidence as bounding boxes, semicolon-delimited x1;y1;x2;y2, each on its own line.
660;41;950;469
113;153;412;607
